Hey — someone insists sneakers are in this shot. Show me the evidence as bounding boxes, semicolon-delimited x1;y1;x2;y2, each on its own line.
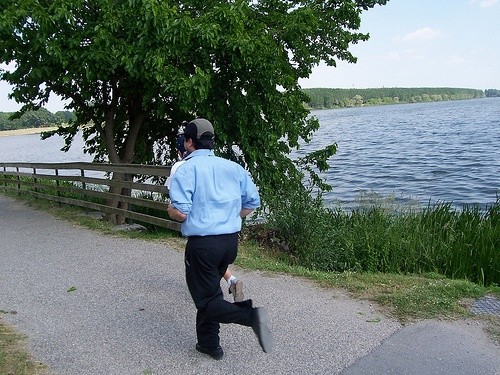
229;277;244;302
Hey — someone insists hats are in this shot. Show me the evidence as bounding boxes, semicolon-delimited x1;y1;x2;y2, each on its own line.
177;118;215;140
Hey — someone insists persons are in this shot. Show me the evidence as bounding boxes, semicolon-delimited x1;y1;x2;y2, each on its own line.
167;117;275;361
168;133;245;304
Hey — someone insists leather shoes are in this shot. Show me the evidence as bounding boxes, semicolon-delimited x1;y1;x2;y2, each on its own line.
251;307;271;354
196;342;222;360
